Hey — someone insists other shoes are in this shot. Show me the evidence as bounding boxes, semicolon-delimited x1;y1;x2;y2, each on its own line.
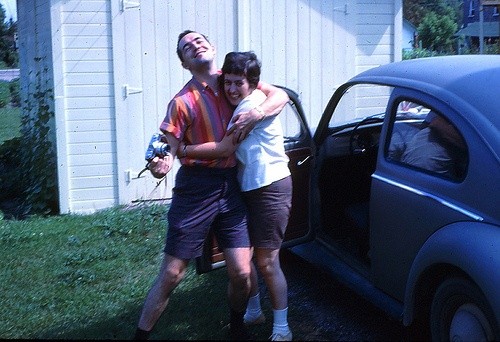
269;327;292;342
221;312;266;331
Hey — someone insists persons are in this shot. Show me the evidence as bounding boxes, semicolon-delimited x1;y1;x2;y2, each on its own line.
177;50;293;342
134;29;290;342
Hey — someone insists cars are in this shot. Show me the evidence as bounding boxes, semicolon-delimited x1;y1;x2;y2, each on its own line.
195;54;500;342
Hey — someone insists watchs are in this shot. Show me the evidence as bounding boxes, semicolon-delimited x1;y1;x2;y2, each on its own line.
182;144;188;158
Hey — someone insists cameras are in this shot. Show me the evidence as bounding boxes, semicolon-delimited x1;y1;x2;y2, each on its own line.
145;133;171;162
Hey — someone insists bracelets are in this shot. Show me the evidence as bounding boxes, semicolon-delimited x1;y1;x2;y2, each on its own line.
254;107;266;123
